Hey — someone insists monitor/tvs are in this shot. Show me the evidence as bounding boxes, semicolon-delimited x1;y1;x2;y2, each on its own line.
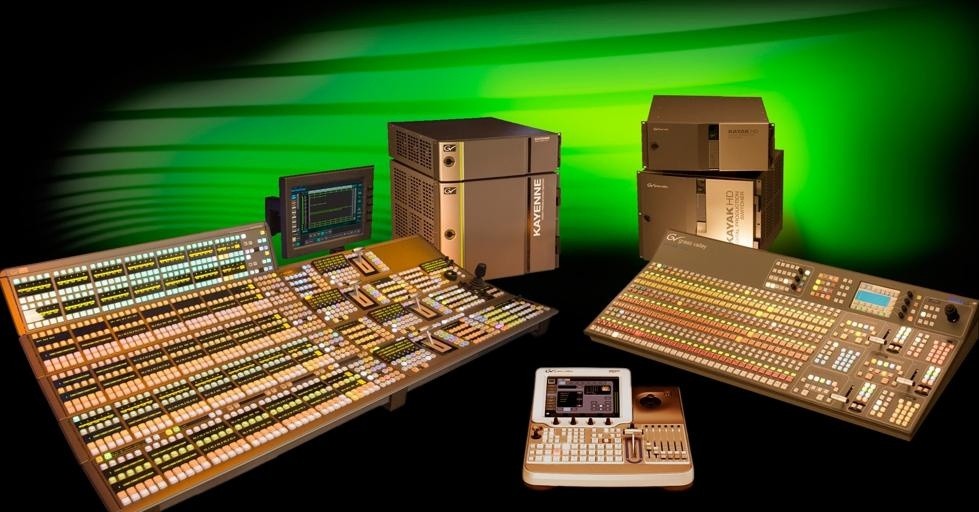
278;165;375;259
